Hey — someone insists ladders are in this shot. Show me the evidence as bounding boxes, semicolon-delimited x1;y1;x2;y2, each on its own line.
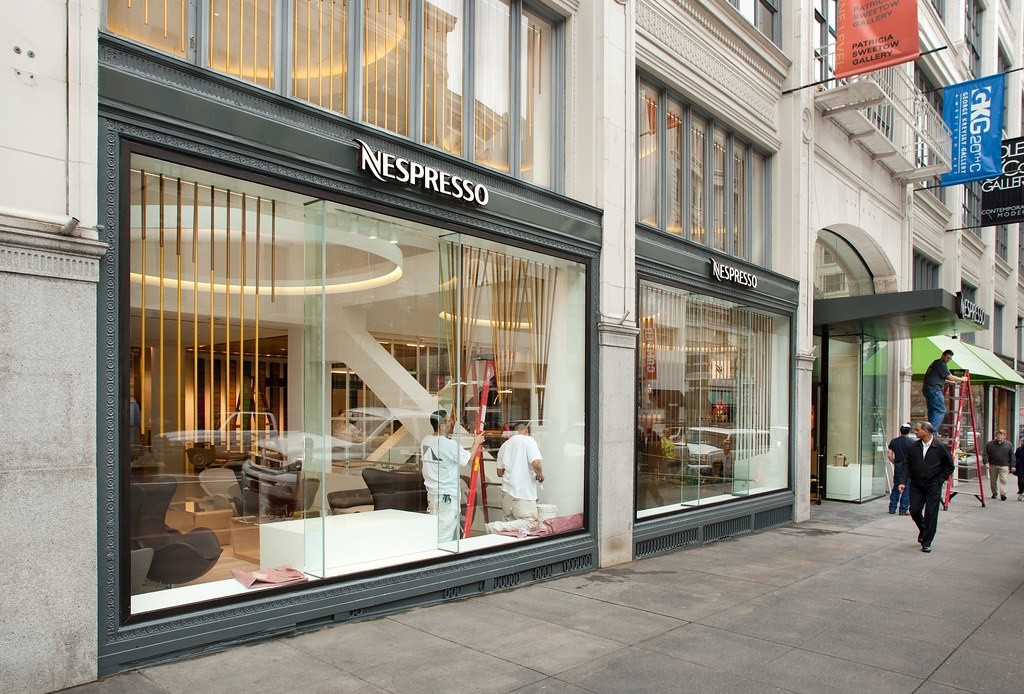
927;368;986;511
449;352;512;541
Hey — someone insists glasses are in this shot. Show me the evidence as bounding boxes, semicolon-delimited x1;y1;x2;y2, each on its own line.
945;356;952;360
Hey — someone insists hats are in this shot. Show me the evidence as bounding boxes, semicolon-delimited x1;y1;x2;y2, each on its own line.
996;429;1006;435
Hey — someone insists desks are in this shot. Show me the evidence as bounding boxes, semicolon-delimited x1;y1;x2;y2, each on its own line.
826;464;873;501
259;509;438;571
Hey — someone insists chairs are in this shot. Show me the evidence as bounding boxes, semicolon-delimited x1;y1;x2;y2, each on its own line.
227;482;243;502
200;468;238;500
130;477;223;584
213;494;242;517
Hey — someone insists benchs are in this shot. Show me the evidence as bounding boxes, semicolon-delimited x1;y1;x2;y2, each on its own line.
361;469;428;512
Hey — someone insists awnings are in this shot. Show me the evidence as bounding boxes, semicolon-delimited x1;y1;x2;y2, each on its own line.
913;336;1024;385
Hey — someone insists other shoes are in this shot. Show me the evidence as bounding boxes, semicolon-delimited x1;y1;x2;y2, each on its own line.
922;545;931;552
918;532;924;543
1018;494;1024;501
1001;495;1006;501
889;510;896;515
899;511;911;516
991;493;997;499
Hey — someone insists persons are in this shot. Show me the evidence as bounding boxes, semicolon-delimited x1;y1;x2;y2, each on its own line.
982;429;1016;501
638;426;733;511
496;421;545;521
1009;439;1024;502
887;423;921;516
922;348;969;439
897;420;955;553
420;410;486;545
337;409;346;426
